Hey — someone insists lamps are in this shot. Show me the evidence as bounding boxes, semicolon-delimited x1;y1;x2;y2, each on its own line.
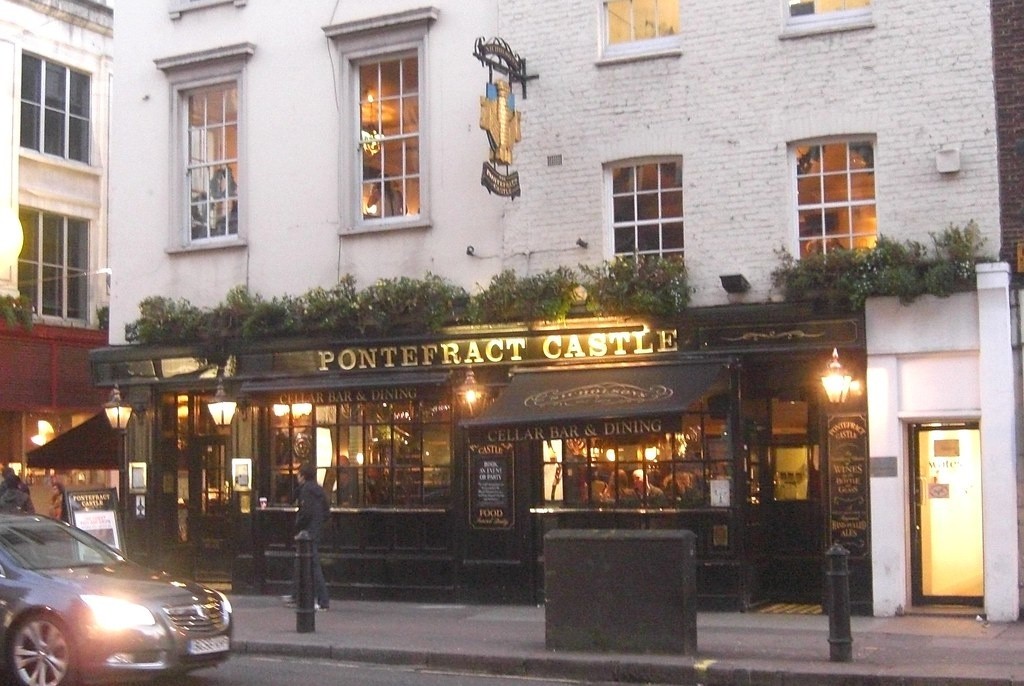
453;364;500;417
576;238;587;248
720;275;752;294
207;378;248;428
103;383;146;433
466;246;474;256
821;348;862;406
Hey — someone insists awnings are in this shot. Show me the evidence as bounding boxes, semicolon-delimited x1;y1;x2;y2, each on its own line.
235;371;453;406
24;408;125;470
456;362;723;428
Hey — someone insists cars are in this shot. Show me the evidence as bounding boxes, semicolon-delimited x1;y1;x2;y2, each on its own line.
0;510;236;686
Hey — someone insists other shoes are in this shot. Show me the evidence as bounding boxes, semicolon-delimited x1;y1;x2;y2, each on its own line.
315;604;328;612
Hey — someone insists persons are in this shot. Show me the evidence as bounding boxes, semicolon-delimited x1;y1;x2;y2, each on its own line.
583;461;706;506
0;474;35;515
49;481;66;520
368;173;409;218
0;467;29;497
281;466;331;611
